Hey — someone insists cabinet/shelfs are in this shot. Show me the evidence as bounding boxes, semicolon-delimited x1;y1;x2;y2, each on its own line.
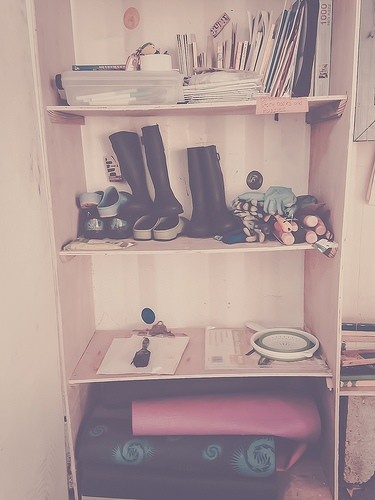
26;0;362;499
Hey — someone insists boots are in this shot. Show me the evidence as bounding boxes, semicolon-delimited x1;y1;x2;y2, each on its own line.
187;147;212;236
140;125;183;214
206;145;243;235
108;131;153;213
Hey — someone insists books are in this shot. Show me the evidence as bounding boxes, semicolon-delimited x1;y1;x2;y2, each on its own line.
171;0;334;103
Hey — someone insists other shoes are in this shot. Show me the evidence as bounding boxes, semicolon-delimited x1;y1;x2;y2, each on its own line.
84;218;105;239
107;218;129;239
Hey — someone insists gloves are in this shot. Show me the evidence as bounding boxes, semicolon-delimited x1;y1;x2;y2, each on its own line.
230;187;297;244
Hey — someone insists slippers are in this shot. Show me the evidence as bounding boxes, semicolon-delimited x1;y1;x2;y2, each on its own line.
134;213;159;240
97;186;131;217
156;217;188;241
80;191;104;206
